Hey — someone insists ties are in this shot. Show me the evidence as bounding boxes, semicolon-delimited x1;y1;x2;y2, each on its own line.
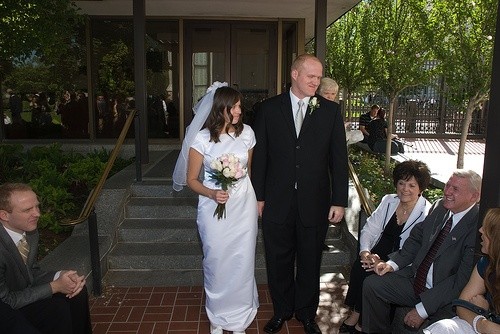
414;214;454;297
18;235;30;265
294;100;304;137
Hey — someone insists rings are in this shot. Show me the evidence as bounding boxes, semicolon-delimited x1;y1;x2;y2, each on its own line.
412;326;414;327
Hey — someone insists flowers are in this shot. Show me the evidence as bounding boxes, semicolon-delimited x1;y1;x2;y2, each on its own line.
308;96;321;115
206;153;248;220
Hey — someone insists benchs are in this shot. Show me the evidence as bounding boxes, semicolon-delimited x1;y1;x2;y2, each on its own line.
391;151;450;189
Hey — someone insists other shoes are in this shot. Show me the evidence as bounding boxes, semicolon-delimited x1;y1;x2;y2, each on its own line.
338;316;362;334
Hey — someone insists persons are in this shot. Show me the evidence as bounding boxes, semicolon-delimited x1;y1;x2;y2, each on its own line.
4;88;177;139
361;169;482;334
249;53;349;334
359;104;404;153
316;77;339;252
423;209;500;334
339;160;432;334
172;79;260;334
367;108;398;153
0;181;92;334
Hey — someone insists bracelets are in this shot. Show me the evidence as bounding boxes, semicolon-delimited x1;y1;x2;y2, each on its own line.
473;315;486;334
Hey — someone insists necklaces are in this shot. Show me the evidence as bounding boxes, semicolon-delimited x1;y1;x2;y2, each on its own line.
401;204;414;214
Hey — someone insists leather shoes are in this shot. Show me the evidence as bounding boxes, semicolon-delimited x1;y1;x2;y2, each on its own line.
295;311;322;334
263;312;293;334
